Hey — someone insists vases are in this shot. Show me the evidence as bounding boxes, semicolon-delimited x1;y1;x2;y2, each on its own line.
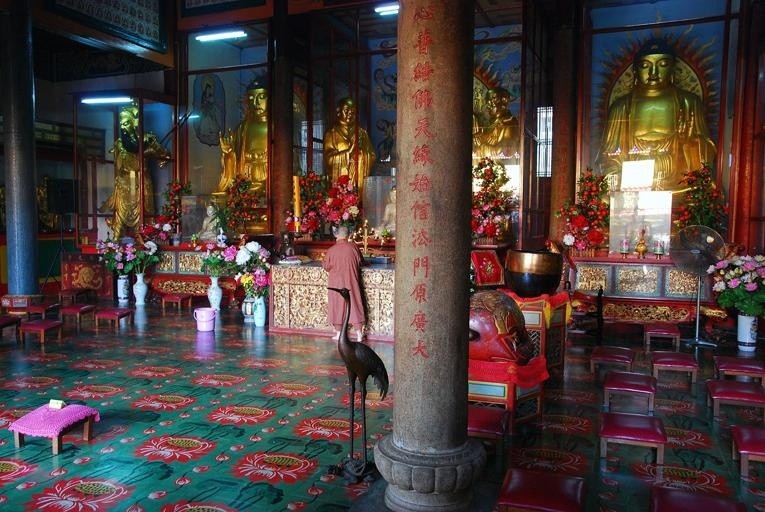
117;276;130;303
133;273;148;305
206;276;266;327
737;310;758;352
332;225;354;237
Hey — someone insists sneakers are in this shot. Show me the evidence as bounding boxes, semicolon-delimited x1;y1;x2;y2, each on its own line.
331;330;367;343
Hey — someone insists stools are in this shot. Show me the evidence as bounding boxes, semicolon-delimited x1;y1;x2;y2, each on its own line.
0;289;134;344
731;424;765;480
591;346;634;388
649;486;745;512
469;402;516;476
162;293;193;310
644;324;681;346
651;350;699;385
604;371;657;414
703;378;765;423
497;466;586;512
599;408;670;479
14;404;93;455
713;355;765;384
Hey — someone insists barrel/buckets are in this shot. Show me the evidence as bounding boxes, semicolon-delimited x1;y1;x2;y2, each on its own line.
192;308;217;331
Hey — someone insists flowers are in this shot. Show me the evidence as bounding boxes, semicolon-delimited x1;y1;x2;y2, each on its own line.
470;205;503;238
562;214;604;251
707;255;765;316
319;176;361;223
284;210;321;235
196;238;272;298
94;223;171;275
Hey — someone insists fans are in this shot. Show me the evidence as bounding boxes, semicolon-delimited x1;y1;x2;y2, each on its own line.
668;225;726;361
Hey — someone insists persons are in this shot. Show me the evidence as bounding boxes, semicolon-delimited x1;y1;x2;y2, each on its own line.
471;87;518;158
322;96;376;204
595;40;715;191
382;187;397;229
217;74;268;193
96;104;171;241
200;82;220;137
323;226;368;344
195;203;223;241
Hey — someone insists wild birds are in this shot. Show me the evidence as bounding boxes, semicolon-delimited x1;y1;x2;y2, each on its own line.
326;287;389;478
